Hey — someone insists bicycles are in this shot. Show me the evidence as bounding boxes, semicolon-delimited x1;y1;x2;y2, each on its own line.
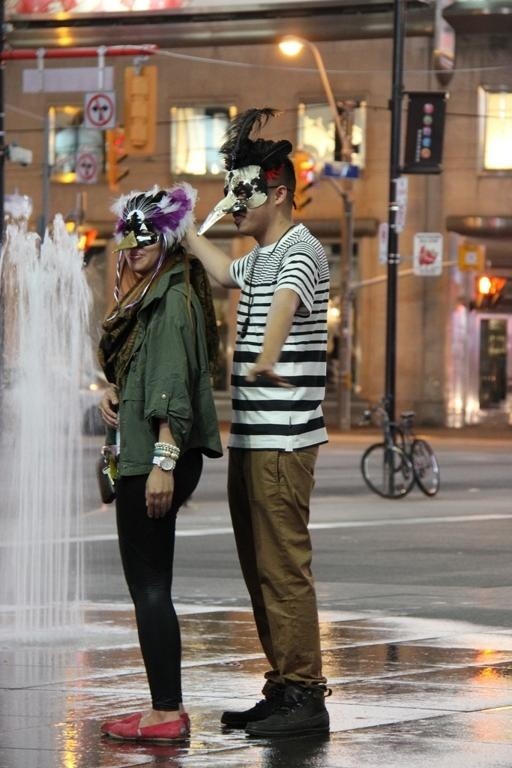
361;393;440;498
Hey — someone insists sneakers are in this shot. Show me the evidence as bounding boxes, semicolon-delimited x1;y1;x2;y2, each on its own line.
99;712;190;741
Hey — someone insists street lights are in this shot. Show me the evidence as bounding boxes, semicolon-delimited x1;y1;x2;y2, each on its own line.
278;34;356;431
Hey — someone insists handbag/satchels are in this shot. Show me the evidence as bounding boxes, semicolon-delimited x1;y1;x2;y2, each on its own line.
98;445;117;504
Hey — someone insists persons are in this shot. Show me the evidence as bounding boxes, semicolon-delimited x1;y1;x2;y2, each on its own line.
178;135;326;739
90;183;223;746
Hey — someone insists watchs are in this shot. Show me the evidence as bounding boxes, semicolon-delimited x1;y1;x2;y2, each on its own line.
151;456;177;471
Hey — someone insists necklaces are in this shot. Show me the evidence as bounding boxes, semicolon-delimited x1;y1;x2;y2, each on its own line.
240;224;298;338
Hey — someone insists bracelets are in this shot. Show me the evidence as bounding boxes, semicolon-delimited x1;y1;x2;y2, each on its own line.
152;442;181;460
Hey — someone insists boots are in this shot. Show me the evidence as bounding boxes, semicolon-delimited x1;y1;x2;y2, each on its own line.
245;683;333;735
221;680;284;724
